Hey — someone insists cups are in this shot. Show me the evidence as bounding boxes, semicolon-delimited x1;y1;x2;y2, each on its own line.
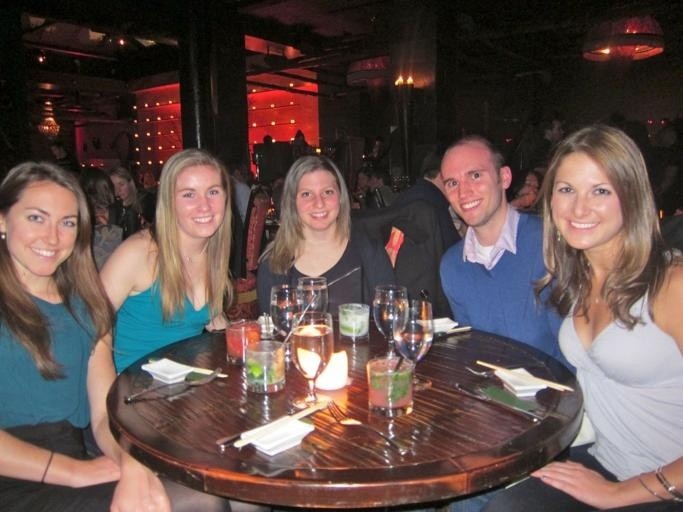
297;277;329;326
246;341;285;394
337;302;370;345
268;285;304;363
372;284;408;359
393;301;433;391
368;359;415;418
224;321;264;364
292;312;332;409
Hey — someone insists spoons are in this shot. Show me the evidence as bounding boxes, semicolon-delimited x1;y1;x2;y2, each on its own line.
451;379;540;424
465;360;547;378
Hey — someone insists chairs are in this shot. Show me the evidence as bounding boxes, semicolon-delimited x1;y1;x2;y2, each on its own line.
225;190;271;319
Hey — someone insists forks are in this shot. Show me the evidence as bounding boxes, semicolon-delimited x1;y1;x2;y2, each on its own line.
122;366;222;404
326;400;408;455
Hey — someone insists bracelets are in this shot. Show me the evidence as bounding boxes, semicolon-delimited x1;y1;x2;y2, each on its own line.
638;465;683;505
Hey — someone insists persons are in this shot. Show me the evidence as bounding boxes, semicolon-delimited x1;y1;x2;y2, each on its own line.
100;147;268;508
439;137;580;377
370;173;398;206
111;132;141;182
48;141;74;169
537;118;564;166
484;126;683;508
0;162;171;507
353;166;385;207
106;168;143;237
223;161;252;227
653;124;683;196
77;167;123;266
510;171;539;208
258;157;396;335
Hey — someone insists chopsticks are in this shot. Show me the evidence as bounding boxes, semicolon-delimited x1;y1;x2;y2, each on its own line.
231;402;330;449
147;357;229;380
473;358;575;393
